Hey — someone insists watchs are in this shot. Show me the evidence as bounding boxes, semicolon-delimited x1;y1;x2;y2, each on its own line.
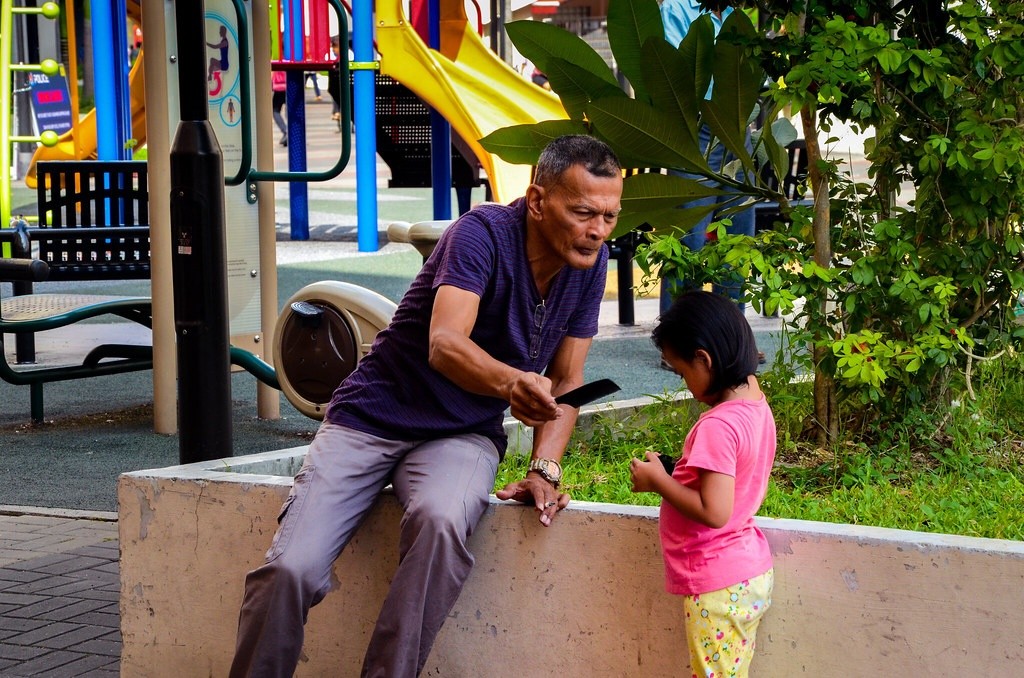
526;458;562;489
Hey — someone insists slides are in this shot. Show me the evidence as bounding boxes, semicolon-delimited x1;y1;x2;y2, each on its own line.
376;24;589;207
24;48;147;189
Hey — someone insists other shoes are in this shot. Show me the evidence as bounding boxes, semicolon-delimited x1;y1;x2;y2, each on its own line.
278;133;288;147
314;96;323;102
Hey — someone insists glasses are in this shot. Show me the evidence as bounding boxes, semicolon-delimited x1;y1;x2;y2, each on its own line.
530;298;549;362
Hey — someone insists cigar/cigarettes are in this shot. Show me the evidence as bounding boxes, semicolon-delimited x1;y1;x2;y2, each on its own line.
534;502;556;511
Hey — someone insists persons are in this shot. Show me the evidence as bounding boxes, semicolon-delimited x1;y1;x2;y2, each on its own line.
658;0;768;372
304;36;322;101
328;38;355;133
272;32;288;147
628;290;777;678
532;67;552;91
127;45;134;66
129;42;142;67
228;136;625;678
